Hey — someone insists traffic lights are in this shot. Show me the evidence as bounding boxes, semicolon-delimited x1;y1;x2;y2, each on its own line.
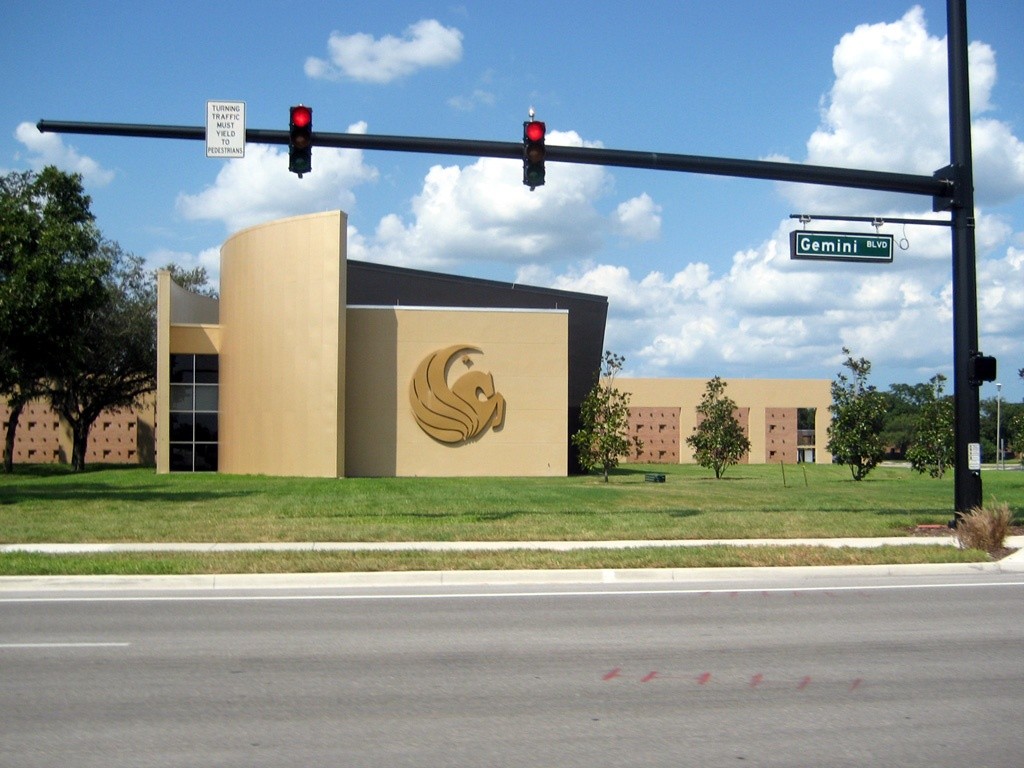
288;103;311;177
970;355;997;383
523;121;545;190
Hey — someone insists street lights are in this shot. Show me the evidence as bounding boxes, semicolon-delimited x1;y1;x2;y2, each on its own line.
996;383;1003;468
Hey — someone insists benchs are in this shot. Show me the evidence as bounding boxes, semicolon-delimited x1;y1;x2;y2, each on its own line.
643;474;665;483
